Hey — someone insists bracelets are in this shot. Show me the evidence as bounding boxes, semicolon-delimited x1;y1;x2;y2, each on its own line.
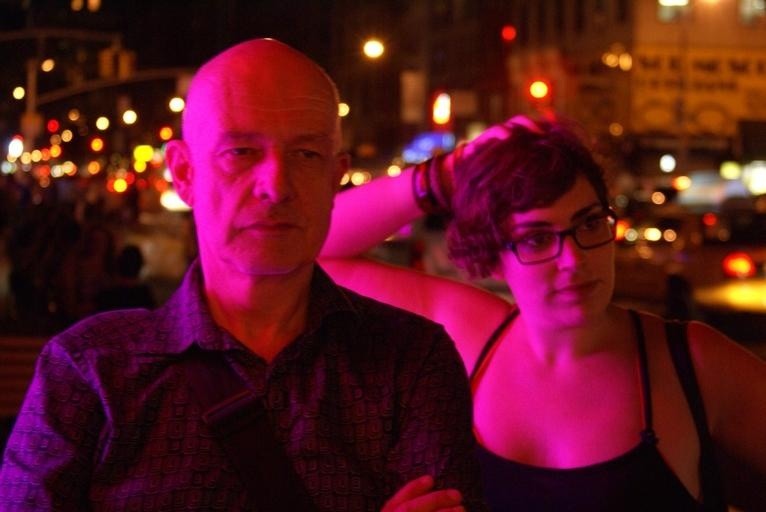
412;146;465;216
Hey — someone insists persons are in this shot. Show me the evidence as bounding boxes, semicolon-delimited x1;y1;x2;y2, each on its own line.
0;182;158;328
0;37;479;511
314;115;766;511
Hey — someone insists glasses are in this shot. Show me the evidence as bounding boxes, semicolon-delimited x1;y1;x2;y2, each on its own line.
490;205;619;267
